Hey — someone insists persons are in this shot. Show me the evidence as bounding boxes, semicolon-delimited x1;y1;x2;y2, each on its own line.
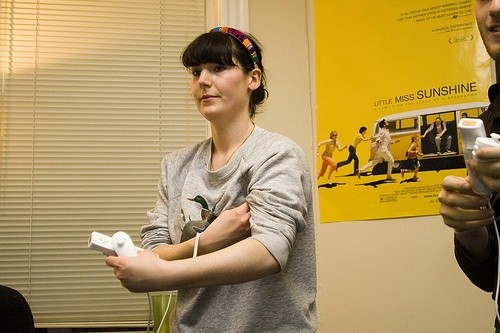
400;135;423;181
317;131;348;185
462;113;467;118
355;118;400;180
106;27;317;333
336;127;371;179
420;118;448;155
438;0;500;333
